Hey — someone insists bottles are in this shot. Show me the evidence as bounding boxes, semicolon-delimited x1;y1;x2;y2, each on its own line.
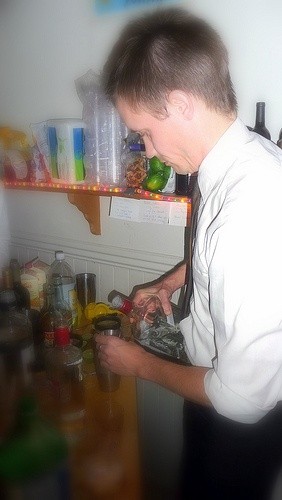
0;258;35;388
111;294;191;363
123;143;149;189
47;319;85;423
46;251;78;319
252;102;272;141
38;276;73;358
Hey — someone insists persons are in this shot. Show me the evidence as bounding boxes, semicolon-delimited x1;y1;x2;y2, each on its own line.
92;5;282;500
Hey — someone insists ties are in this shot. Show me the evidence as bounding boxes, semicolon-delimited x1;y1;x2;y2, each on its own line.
181;172;202;320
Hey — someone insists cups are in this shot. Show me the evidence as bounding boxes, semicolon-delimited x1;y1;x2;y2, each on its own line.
92;328;121;392
76;273;96;309
82;89;128;186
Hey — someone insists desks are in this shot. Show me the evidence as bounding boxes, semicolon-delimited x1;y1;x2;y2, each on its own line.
0;290;144;500
